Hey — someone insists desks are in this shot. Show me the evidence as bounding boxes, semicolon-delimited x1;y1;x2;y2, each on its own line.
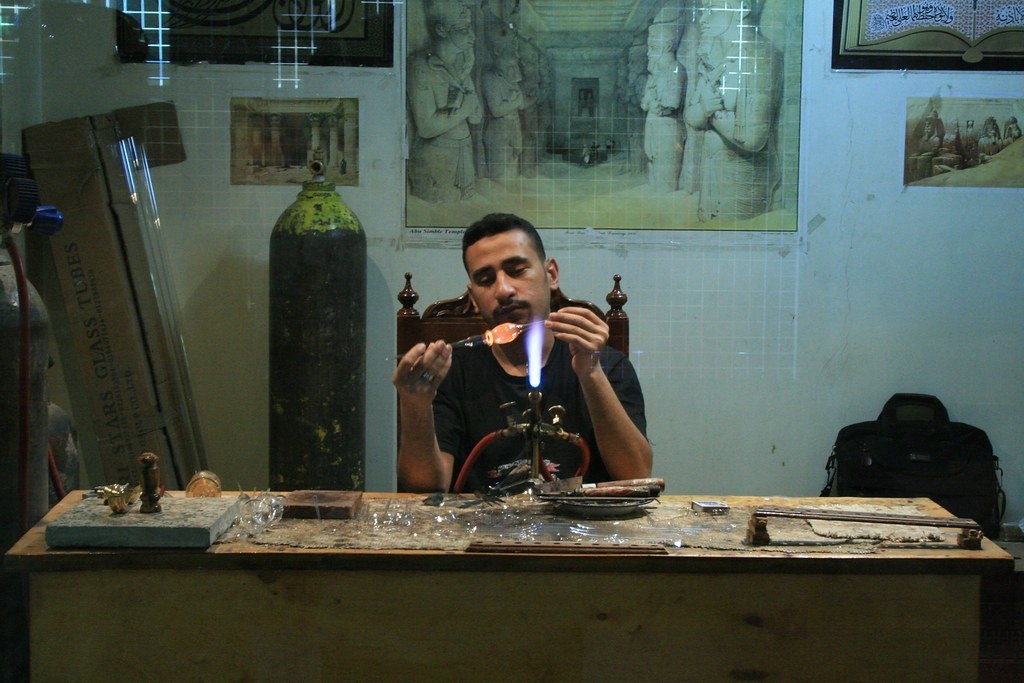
0;489;1014;683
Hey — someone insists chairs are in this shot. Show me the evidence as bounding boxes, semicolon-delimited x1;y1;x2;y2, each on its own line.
395;272;631;494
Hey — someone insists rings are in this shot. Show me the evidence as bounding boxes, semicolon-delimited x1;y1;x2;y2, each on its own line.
422;372;433;381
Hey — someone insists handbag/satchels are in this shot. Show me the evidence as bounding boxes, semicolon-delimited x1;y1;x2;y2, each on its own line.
819;393;1006;539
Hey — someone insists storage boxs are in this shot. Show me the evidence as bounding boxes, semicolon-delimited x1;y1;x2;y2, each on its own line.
20;115;195;490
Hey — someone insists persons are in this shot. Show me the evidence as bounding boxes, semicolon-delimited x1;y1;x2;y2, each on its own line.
393;214;653;493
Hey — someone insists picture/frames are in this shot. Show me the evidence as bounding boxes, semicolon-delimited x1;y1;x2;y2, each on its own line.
115;0;394;69
830;0;1024;76
